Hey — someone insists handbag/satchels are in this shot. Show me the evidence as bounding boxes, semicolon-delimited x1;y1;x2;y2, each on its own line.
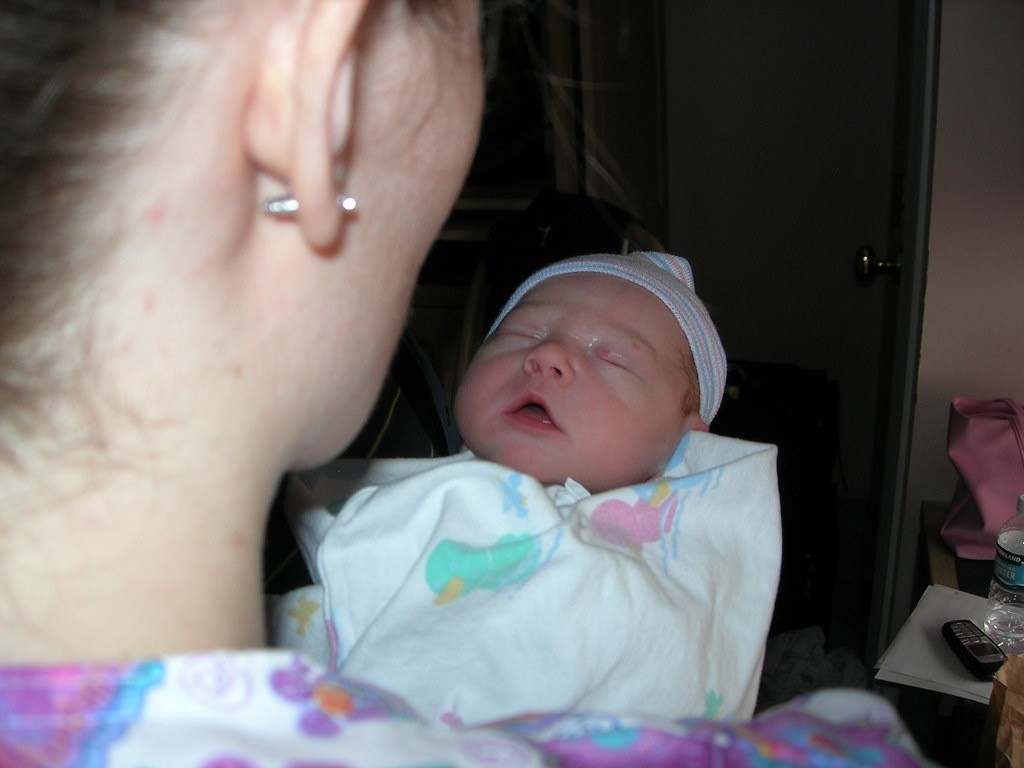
939;394;1024;560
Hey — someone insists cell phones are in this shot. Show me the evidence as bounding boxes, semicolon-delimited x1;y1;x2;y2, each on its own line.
943;619;1008;682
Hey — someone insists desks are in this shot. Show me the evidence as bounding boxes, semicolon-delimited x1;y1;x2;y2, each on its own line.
438;186;544;405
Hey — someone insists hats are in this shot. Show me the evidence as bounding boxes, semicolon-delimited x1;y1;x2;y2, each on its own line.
481;252;728;432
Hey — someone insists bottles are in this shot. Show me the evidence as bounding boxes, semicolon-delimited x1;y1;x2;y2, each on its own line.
985;493;1024;655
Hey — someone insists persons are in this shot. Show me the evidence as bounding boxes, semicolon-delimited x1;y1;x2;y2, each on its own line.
1;1;925;768
